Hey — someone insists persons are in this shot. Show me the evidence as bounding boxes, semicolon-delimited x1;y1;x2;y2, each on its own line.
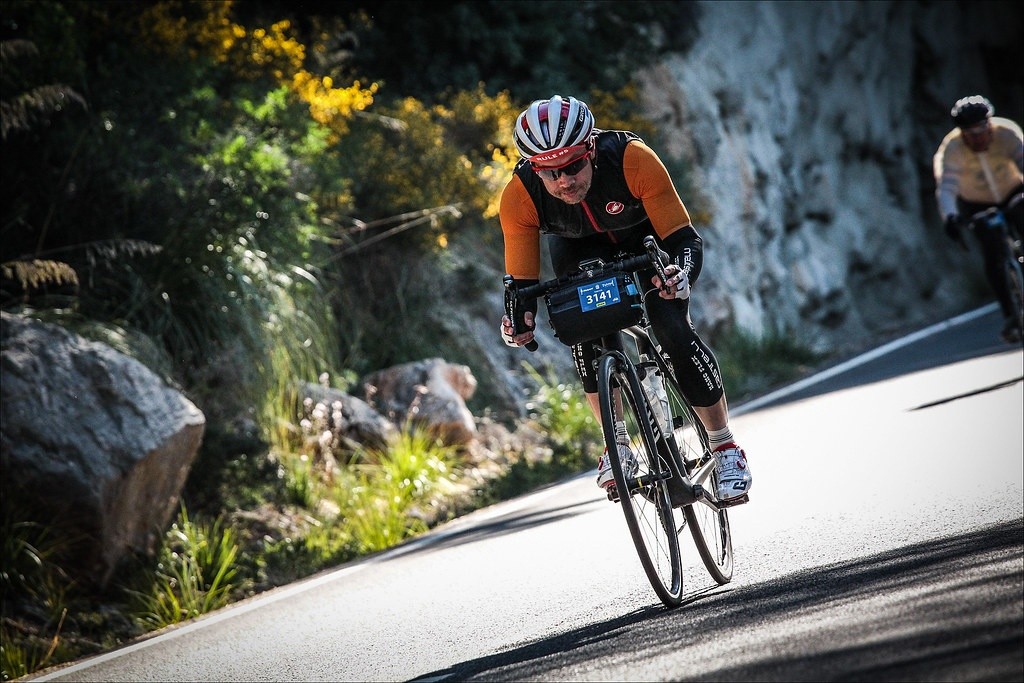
499;94;752;502
932;95;1024;344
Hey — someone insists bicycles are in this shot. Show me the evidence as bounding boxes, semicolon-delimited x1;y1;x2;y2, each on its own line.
950;185;1024;343
504;234;733;607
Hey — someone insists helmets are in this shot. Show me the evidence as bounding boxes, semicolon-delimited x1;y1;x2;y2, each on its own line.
951;95;994;128
512;95;595;162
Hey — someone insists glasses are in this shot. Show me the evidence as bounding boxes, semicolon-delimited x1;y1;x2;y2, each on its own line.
532;146;593;181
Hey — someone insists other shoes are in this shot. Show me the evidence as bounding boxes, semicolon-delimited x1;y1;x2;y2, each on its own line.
1005;326;1020;342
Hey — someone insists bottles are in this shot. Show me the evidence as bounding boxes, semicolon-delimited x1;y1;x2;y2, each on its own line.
635;354;674;439
636;363;668;432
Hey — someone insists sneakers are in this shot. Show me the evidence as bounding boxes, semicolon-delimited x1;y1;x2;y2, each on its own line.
596;444;639;488
712;441;751;500
